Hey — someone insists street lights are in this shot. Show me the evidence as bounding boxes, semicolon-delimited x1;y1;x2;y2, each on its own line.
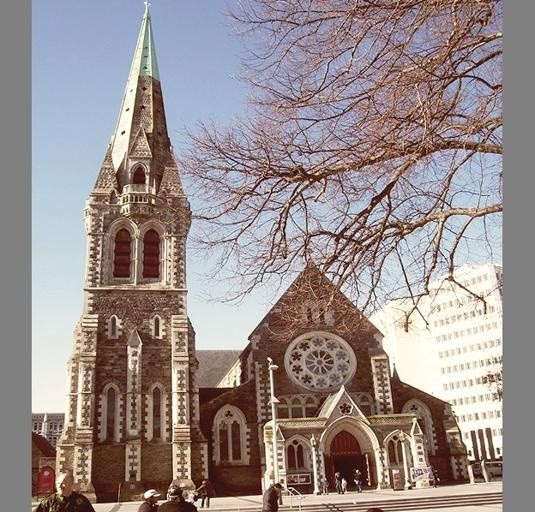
265;355;284;508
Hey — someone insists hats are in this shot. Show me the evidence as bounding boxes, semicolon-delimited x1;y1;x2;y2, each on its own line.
143;489;162;500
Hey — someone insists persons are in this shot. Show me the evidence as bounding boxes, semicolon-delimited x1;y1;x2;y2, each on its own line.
353;468;363;493
340;477;348;493
137;488;161;512
430;467;439;488
261;483;283;511
156;485;198;511
321;477;330;495
195;480;212;508
35;467;95;510
335;471;345;493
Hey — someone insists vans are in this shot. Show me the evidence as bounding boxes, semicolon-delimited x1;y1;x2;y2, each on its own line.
473;461;503;478
